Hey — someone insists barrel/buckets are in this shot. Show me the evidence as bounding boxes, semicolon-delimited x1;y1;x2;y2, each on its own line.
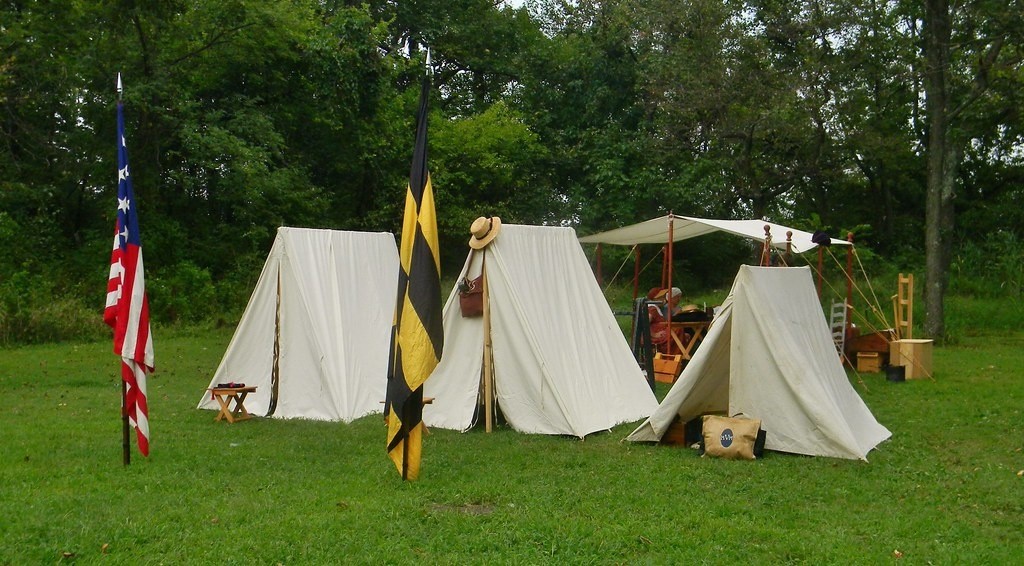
886;365;906;382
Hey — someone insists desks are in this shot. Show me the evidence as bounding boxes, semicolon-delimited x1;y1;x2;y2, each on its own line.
659;320;710;361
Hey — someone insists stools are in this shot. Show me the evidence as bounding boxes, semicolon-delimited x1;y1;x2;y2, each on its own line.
206;386;258;423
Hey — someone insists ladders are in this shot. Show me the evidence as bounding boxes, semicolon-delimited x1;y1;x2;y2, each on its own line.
828;297;848;357
898;273;913;339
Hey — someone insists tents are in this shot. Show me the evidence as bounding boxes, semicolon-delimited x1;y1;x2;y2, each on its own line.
576;213;854;374
620;263;894;464
195;224;400;426
421;222;661;442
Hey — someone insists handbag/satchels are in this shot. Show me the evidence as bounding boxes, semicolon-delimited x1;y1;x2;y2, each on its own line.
458;276;483;317
698;413;767;461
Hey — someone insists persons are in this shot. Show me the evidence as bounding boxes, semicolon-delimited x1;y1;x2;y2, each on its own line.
640;286;701;367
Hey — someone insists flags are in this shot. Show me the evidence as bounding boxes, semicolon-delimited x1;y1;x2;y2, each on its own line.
381;73;446;486
100;97;156;459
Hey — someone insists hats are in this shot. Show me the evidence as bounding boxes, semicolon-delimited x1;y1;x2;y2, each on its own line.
647;287;668;300
468;216;501;250
665;287;682;301
